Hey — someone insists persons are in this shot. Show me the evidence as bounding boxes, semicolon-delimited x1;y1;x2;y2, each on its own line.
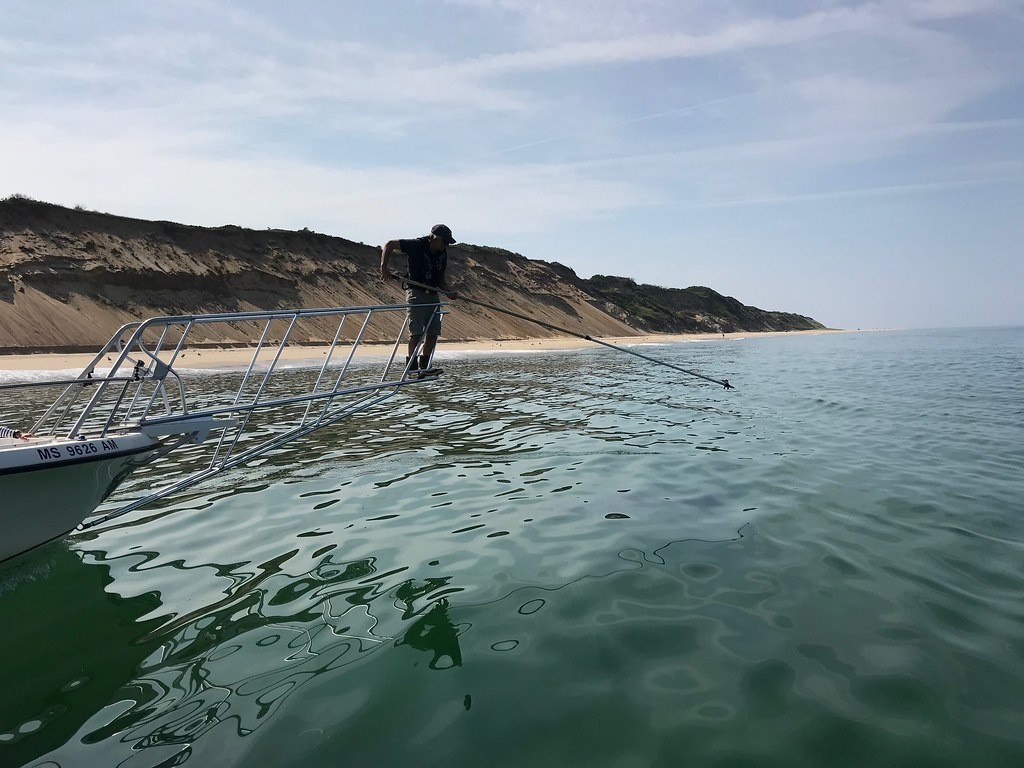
381;224;458;380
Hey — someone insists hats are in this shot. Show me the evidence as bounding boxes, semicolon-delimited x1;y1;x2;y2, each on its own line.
431;224;456;244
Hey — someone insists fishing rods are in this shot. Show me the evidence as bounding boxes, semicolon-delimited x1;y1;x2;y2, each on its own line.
381;269;735;391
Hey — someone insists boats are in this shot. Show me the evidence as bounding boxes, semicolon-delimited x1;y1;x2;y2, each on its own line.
0;302;450;563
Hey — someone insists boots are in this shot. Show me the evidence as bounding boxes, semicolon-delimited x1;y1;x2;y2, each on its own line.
405;356;424;379
419;355;444;375
